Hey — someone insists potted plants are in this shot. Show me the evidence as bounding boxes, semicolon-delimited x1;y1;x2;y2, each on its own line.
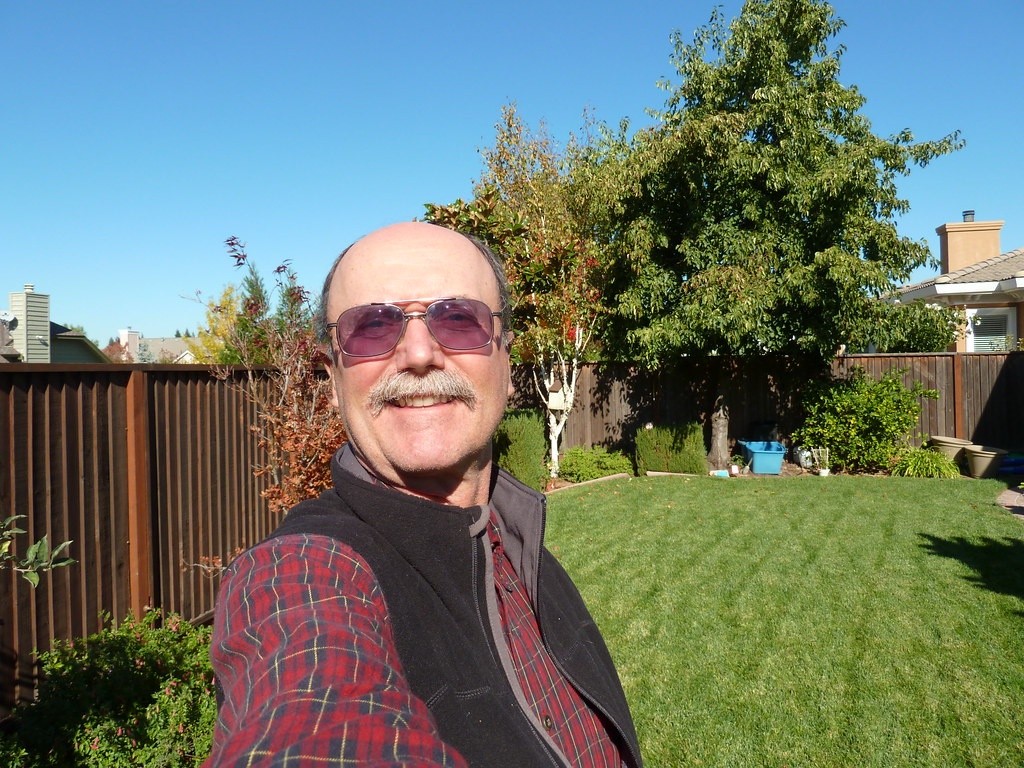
814;456;830;476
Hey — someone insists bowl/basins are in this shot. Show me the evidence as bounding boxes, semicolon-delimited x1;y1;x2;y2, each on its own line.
964;445;1009;479
931;435;973;462
818;468;830;477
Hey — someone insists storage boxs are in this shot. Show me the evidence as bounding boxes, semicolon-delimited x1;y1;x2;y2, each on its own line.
737;433;789;474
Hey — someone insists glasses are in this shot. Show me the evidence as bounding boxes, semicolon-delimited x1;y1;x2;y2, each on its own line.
326;300;502;358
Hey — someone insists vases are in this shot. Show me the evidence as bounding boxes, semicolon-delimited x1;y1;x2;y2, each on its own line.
930;435;1010;478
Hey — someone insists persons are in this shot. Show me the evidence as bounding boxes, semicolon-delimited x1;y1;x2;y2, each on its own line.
203;222;644;767
